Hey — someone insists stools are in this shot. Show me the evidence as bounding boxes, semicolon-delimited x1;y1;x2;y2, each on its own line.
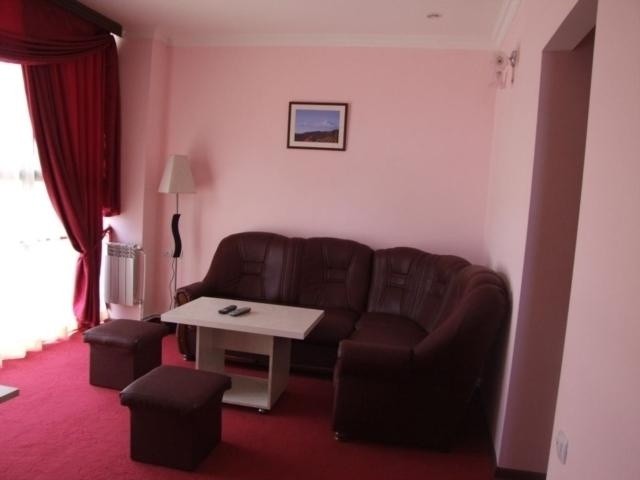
120;364;232;470
83;319;163;390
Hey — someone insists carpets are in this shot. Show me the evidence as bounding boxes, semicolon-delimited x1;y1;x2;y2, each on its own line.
0;318;492;480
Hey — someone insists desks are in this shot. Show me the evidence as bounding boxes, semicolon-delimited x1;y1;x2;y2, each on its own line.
161;296;326;414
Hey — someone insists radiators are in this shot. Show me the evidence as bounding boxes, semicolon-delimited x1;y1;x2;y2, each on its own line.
105;243;139;308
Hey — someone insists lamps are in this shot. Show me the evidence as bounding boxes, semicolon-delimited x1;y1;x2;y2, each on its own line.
159;154;197;288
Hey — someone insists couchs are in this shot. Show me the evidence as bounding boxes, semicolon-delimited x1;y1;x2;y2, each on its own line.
274;235;375;376
331;246;509;441
177;232;285;366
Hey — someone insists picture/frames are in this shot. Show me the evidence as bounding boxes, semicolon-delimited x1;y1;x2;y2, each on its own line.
287;100;347;151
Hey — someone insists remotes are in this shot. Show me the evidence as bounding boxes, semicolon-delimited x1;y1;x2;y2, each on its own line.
229;307;250;316
218;304;237;314
